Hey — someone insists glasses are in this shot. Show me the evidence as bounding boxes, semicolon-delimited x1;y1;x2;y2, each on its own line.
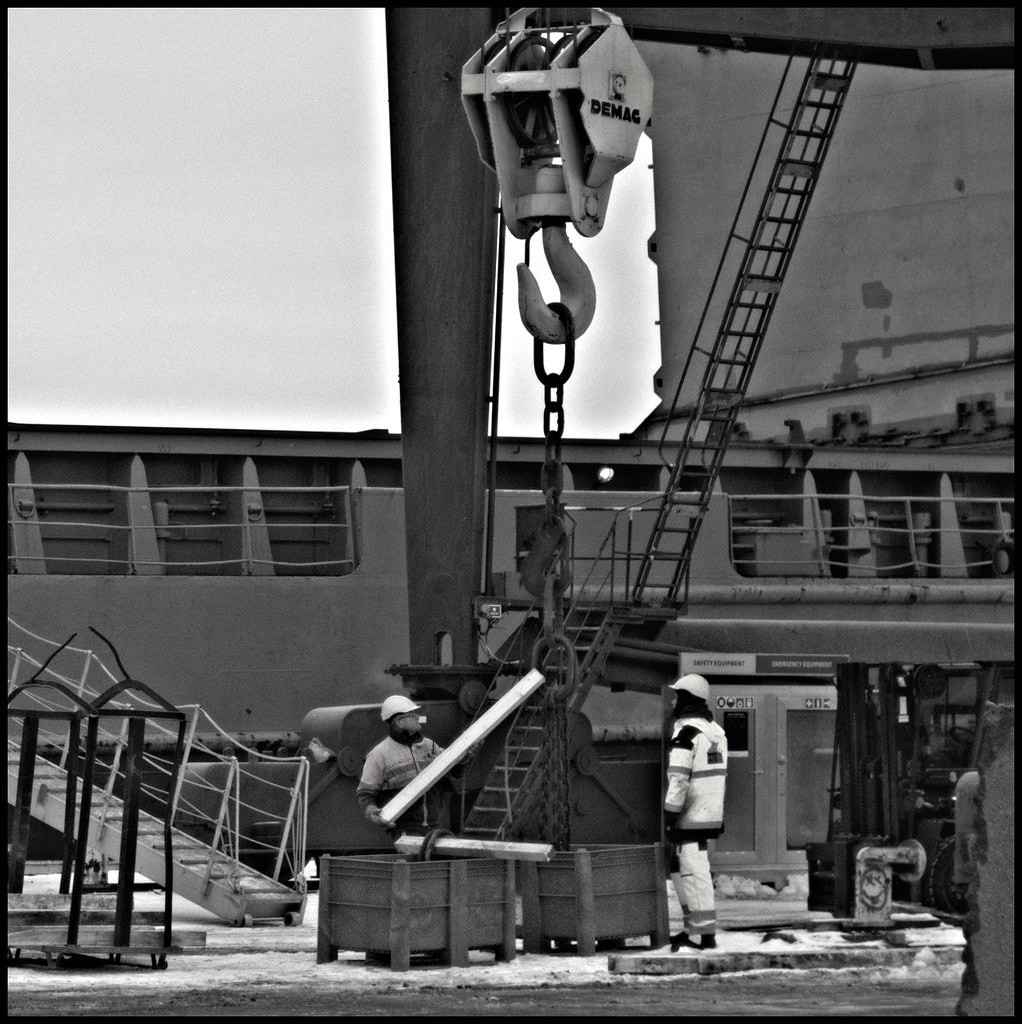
398;711;416;720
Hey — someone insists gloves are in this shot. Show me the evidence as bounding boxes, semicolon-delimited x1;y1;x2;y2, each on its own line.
663;810;679;828
364;805;396;828
460;740;484;766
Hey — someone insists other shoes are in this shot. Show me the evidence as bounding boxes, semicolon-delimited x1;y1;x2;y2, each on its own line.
669;931;716;949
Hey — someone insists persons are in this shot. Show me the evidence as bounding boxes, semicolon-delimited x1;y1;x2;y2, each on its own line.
952;760;989;1012
664;674;728;950
357;695;483;959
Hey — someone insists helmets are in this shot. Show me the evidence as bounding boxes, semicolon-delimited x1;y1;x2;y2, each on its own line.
668;674;709;700
381;695;422;722
